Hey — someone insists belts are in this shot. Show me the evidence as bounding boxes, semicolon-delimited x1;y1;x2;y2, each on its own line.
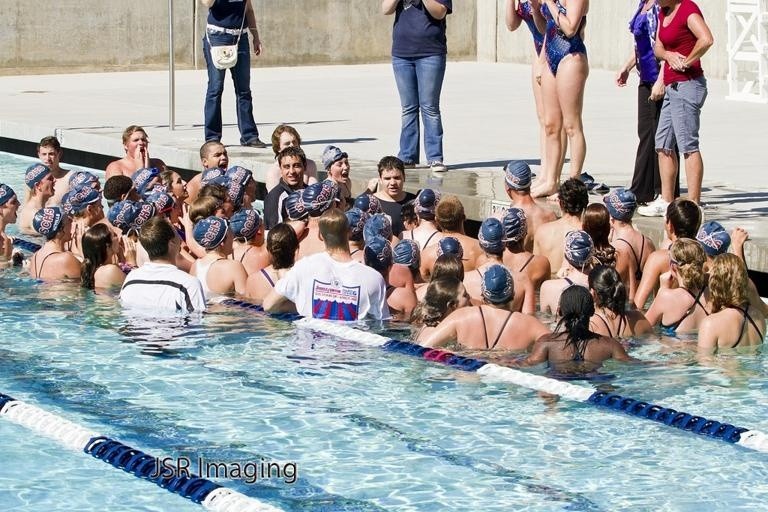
207;23;248;36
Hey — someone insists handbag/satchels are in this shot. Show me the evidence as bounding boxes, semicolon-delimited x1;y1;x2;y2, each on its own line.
210;45;238;70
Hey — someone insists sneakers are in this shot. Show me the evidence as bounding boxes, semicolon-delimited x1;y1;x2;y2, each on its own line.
249;138;266;148
430;160;447;172
637;194;671;217
403;161;415;169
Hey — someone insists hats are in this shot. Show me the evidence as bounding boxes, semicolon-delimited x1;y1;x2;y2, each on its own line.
193;165;260;250
564;231;593;265
0;163;101;240
285;179;337;220
344;194;419;267
413;188;440;214
480;264;514;303
437;236;464;259
504;160;532;191
321;145;348;170
605;189;637;221
478;208;527;251
696;221;731;256
107;167;174;230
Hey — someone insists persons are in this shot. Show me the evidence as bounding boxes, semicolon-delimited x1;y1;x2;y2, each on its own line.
529;0;589;199
636;0;715;219
202;0;266;148
381;0;453;172
505;0;568;191
615;0;680;205
7;124;768;374
